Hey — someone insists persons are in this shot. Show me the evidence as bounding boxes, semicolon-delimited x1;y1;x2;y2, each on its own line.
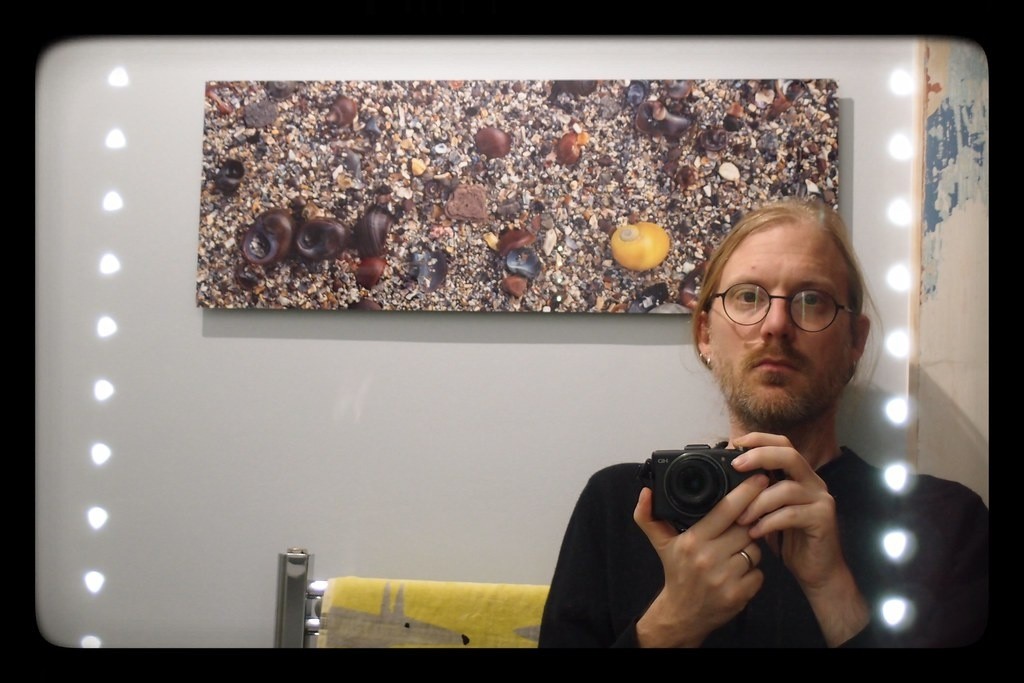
537;201;988;653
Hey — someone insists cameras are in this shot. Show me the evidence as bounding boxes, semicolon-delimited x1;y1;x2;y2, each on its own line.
651;441;767;526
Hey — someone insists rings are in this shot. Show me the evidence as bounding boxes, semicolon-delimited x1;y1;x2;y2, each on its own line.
739;549;753;574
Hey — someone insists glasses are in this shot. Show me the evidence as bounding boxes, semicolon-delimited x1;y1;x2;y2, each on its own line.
711;282;854;333
